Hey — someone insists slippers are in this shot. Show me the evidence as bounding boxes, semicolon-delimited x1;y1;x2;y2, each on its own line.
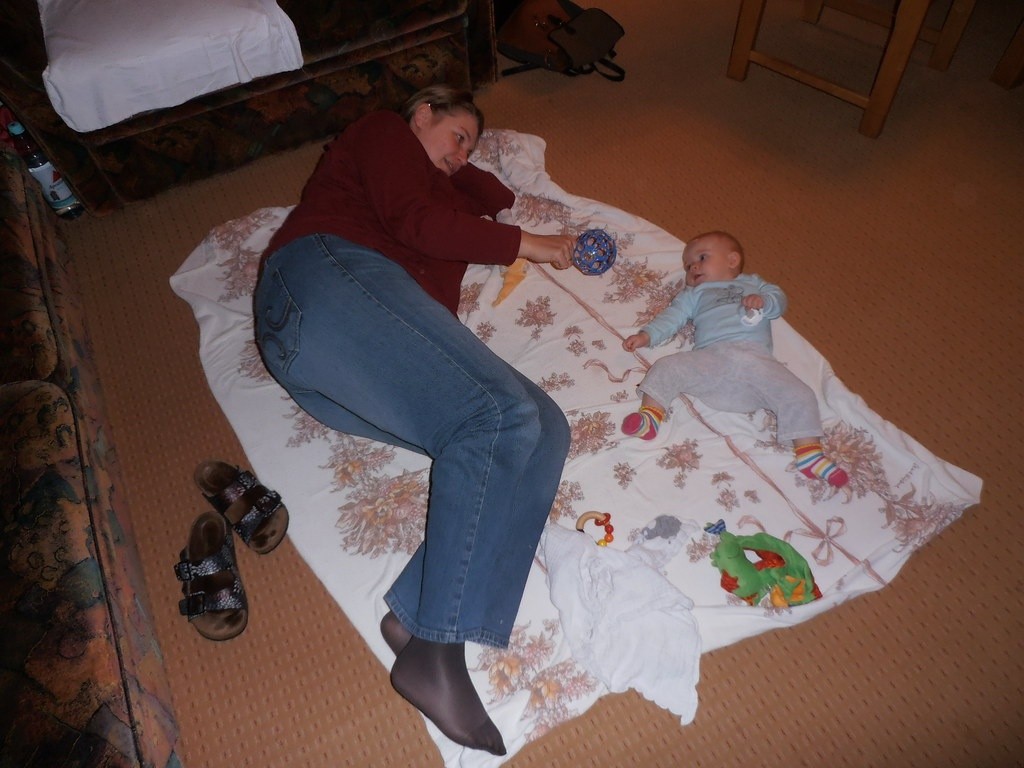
173;511;248;641
194;460;289;555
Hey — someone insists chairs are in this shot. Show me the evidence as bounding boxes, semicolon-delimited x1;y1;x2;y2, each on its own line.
719;1;982;139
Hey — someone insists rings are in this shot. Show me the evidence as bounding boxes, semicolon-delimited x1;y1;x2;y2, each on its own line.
567;258;572;261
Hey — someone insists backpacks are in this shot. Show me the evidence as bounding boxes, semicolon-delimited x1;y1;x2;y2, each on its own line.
494;0;626;83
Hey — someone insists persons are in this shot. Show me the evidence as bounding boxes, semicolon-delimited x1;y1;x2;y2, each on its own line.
252;85;590;756
621;231;848;489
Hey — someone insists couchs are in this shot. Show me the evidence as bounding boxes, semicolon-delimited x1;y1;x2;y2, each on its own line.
0;0;501;217
0;101;190;768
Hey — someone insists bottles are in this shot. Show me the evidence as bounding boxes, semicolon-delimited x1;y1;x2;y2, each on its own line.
7;122;84;220
